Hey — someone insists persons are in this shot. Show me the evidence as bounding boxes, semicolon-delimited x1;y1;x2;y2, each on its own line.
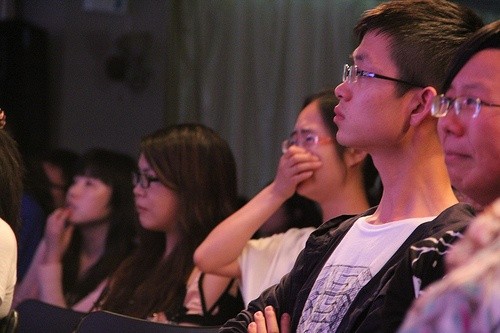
98;123;238;327
10;150;133;313
192;91;378;310
354;20;500;333
217;0;486;333
0;108;17;319
